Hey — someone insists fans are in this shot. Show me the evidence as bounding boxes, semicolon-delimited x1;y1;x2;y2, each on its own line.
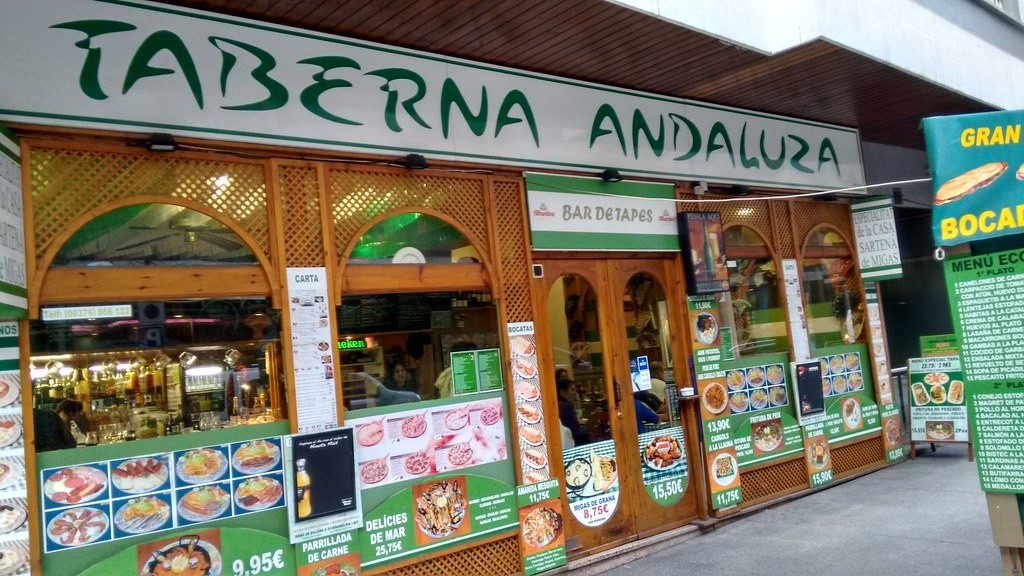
115;217;243;251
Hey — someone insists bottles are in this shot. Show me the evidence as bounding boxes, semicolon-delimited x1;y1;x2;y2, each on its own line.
87;404;132;427
295;457;312;517
136;409;186;439
184;373;224;392
229;396;240;421
253;393;267;413
32;367;164;408
85;431;98;446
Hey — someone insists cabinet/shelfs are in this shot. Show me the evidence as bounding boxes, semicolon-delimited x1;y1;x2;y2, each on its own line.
339;347;385;410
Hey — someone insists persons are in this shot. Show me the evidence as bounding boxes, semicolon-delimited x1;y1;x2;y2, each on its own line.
555;361;671;451
434;341;478;398
382;361;420;392
30;399;90;453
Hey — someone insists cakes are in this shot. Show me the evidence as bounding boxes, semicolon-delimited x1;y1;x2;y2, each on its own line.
717;457;732;476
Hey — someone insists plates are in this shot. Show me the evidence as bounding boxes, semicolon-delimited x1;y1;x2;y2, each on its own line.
416;486;466;538
138;538;223;576
0;419;22;449
910;371;965;406
0;378;20;408
43;441;283;547
752;422;783;452
711;453;738;486
694;311;718;345
0;503;27;535
926;423;953;440
885;418;900;445
808;440;830;469
0;547;26;576
868;305;892;404
510;338;547;484
727;366;787;413
643;437;683;470
572;454;617;497
392;246;427;263
522;507;558;548
820;355;863;396
0;459;22;490
702;382;728;415
842;398;861;428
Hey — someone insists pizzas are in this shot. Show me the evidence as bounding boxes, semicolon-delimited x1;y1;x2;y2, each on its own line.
357;422;383;445
361;461;388;483
480;409;500;425
404;453;430;474
448;446;472;464
445;410;468;430
401;417;426;437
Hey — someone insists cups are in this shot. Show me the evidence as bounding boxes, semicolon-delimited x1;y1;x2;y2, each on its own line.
99;421;129;444
178;351;198;370
102;356;119;374
128;355;147;371
44;359;63;379
190;411;222;431
656;440;670;453
223;348;241;366
153;353;172;371
166;363;182;411
565;458;592;490
